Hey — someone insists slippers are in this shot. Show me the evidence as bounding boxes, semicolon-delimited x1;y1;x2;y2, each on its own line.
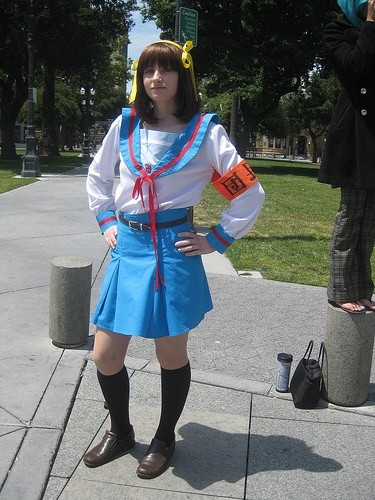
359;298;375;311
328;297;366;314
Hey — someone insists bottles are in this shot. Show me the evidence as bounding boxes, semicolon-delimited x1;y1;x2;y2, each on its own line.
275;352;293;392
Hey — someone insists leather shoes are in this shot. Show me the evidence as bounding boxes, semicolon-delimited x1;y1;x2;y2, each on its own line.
136;433;174;478
84;422;135;467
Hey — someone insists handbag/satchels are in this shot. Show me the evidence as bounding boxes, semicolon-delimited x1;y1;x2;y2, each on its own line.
290;340;328;408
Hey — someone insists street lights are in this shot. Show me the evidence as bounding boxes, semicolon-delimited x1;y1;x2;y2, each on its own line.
80;86;96;162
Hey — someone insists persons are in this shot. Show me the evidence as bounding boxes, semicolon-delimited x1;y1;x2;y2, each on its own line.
319;0;375;314
83;40;266;478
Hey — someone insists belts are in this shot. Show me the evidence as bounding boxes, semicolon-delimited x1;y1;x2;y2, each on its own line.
118;212;187;232
